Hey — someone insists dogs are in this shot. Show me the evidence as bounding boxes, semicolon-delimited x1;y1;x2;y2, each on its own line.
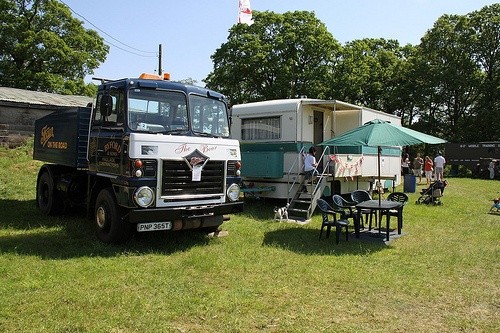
272;203;290;223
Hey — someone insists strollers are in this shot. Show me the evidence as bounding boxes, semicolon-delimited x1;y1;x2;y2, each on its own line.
414;180;447;208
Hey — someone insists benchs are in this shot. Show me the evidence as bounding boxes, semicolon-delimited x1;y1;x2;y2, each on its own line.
129;111;187;125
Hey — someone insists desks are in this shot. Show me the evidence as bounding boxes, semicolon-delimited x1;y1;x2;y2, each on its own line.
355;199;403;241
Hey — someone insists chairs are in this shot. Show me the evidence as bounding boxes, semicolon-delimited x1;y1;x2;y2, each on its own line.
381;192;408;229
351;190;377;229
332;194;364;232
316;199;350;245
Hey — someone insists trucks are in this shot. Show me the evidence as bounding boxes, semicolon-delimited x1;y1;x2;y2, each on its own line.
224;97;403;221
32;74;246;246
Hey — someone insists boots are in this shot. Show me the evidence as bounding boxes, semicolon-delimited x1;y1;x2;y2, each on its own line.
303;185;308;192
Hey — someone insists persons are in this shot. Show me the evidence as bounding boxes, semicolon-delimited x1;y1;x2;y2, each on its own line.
434;151;446;181
401;152;411;175
413;153;424;184
489;160;497;180
425;156;434;185
303;147;319;193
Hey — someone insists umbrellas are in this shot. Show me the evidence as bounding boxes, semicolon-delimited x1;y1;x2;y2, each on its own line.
317;118;448;235
